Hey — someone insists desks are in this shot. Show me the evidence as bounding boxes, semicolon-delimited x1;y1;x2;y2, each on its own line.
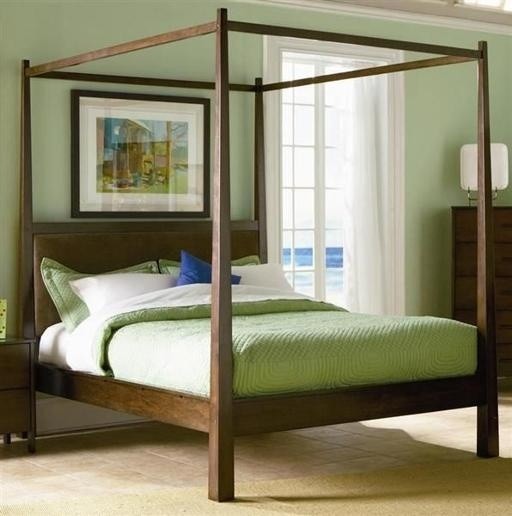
0;336;35;454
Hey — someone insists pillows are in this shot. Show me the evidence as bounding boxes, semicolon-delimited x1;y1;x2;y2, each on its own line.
159;255;261;274
176;252;241;287
69;273;175;314
40;255;161;332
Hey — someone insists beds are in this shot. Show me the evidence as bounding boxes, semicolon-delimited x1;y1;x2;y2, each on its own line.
18;7;494;503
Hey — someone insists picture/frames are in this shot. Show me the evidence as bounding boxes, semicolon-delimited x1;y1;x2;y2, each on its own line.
68;88;210;220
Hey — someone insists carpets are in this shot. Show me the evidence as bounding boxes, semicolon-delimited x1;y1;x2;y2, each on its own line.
0;453;511;516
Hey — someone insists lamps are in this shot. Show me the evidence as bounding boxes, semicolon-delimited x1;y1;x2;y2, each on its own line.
456;143;511;209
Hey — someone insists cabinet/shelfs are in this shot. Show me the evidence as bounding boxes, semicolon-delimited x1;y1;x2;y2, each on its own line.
452;203;512;393
